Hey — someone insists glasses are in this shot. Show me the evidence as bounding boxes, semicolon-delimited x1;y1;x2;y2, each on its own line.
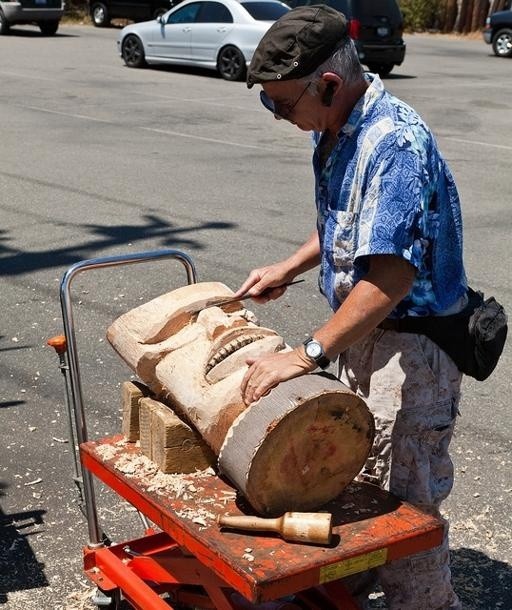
259;72;325;122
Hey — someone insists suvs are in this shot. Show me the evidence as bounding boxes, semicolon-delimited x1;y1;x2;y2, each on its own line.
483;6;512;59
281;1;406;80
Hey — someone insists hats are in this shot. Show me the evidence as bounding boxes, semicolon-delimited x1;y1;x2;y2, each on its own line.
245;3;351;89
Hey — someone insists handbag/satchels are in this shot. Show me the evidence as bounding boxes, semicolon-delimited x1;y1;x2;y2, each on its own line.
458;289;508;381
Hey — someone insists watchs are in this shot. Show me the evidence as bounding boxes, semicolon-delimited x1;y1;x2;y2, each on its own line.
301;336;331;371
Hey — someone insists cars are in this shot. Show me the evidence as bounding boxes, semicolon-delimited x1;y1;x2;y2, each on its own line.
88;1;181;29
116;1;292;83
0;0;66;36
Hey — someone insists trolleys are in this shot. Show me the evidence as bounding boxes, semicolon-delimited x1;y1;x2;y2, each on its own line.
50;249;445;609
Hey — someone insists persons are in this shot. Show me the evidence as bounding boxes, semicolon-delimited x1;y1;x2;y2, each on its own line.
231;4;469;516
